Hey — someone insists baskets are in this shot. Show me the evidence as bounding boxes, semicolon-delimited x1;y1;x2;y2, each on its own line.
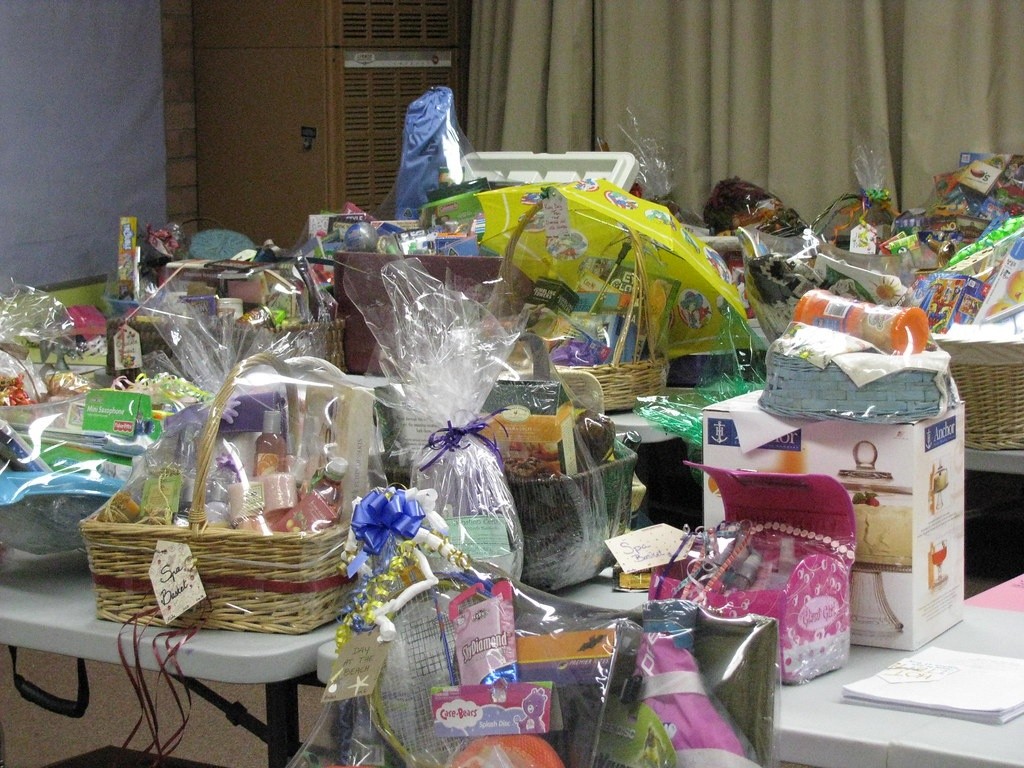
928;337;1024;450
485;195;671;412
759;344;942;423
105;313;346;382
80;489;355;636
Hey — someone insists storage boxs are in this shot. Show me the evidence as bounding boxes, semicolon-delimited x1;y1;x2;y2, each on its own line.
525;278;579;324
914;270;985;337
701;386;966;652
332;248;533;373
567;311;645;361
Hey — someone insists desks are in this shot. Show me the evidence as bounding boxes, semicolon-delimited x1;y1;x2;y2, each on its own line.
607;408;683;443
0;566;1023;766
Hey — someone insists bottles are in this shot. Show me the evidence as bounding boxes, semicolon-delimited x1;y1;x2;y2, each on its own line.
252;409;287;478
308;457;350;523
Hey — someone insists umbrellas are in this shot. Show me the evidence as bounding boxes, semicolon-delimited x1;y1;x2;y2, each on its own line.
473;178;758;361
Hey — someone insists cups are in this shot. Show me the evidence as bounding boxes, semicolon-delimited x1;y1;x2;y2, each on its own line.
792;289;931;356
